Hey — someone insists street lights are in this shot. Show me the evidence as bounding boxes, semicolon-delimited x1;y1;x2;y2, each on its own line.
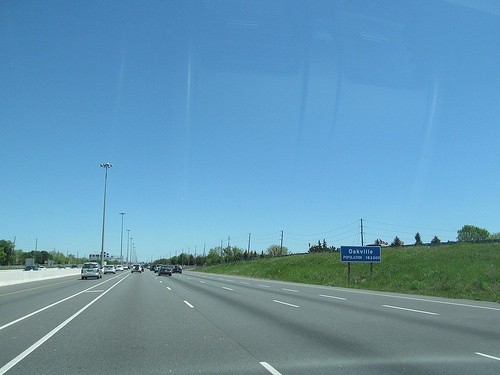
120;211;126;265
126;229;138;267
99;163;113;272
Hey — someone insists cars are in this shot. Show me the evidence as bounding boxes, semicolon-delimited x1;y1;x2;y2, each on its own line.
171;265;182;273
157;265;171;276
123;263;164;273
116;264;123;271
80;261;103;280
103;264;116;274
